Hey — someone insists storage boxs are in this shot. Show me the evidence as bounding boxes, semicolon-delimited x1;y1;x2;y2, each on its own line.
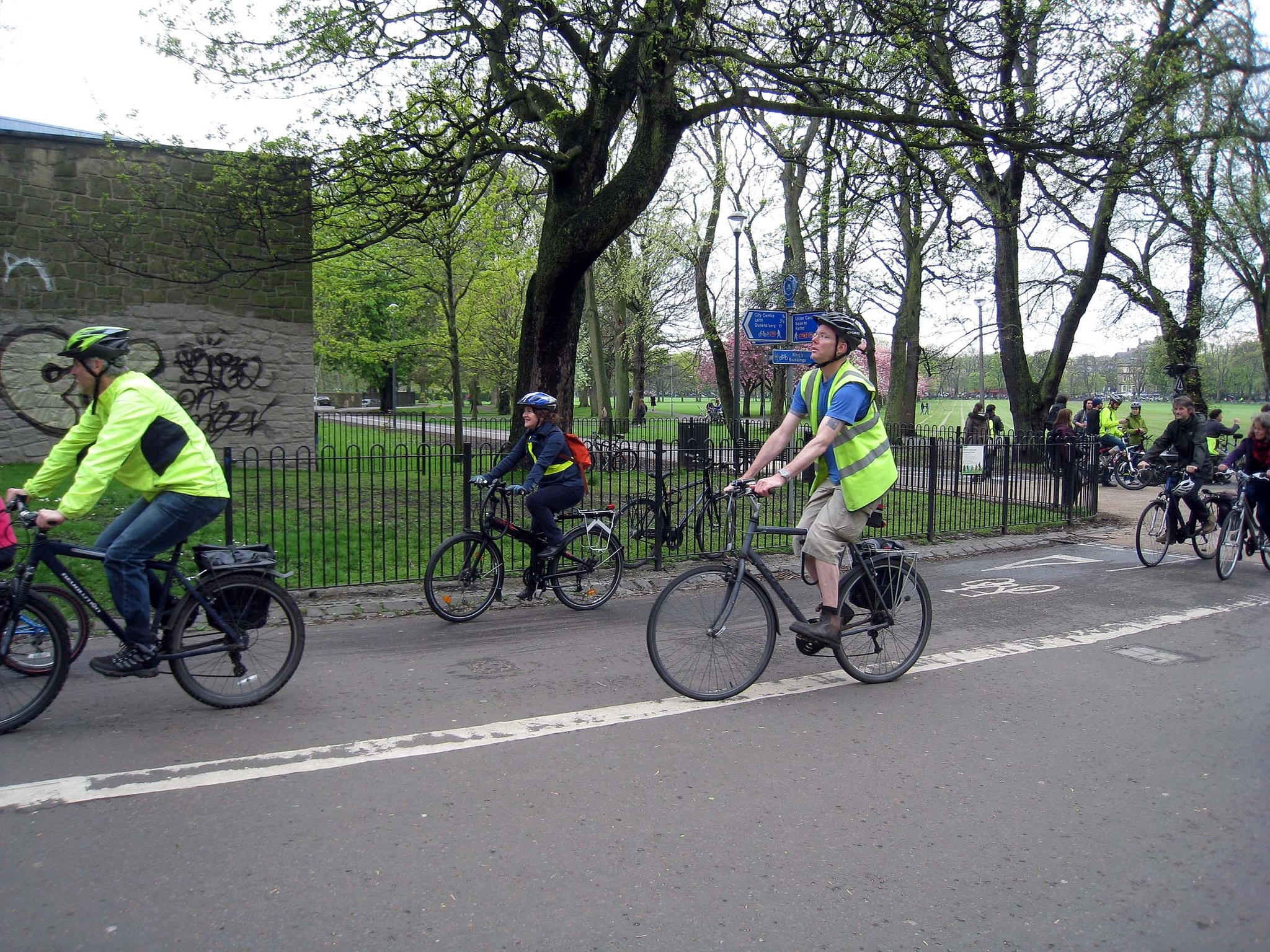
195;543;275;630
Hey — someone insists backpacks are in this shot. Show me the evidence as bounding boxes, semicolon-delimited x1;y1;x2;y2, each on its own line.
1046;425;1061;454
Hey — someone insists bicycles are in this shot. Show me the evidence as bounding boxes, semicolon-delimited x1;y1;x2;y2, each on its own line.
1042;428;1246;491
0;498;306;734
1135;463;1231;567
0;584;89;675
697;406;716;423
584;432;638;473
424;478;625;622
1212;464;1270;580
646;479;933;702
612;453;736;569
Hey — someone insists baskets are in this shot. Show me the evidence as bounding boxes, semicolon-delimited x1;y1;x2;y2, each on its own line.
849;537;904;611
188;543;277;629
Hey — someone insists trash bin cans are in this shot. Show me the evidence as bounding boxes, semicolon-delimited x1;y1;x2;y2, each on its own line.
677;420;709;468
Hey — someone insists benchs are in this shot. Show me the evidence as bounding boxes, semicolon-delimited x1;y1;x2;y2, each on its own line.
734;438;790;476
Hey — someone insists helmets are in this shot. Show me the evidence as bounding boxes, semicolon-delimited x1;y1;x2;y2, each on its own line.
55;325;132;365
1110;392;1124;404
812;312;865;351
1170;475;1195;497
1130;401;1142;408
516;391;557;412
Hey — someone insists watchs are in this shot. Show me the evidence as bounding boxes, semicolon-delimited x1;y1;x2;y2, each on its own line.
777;469;791;483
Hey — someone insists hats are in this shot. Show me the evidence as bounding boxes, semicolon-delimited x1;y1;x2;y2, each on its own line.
1092;397;1102;408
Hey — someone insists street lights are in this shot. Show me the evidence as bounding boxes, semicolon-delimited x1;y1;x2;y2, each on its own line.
727;211;748;424
387;304;401;413
974;298;986;411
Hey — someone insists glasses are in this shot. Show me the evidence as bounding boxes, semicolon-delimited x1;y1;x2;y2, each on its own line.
812;331;846;342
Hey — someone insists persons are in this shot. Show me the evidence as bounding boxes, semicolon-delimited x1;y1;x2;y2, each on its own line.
468;392;584;600
629;392;657;427
960;403;1002;483
1;496;18;571
722;313;898;650
920;401;931;416
1044;392;1269;544
6;327;233;678
713;400;723;420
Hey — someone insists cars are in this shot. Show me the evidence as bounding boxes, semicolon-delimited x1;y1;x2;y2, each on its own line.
314;396;331;407
1090;391;1163;401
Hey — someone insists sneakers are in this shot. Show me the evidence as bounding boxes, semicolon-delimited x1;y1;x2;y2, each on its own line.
971;474;978;482
1158;530;1177;545
91;640;161;677
1203;511;1217;534
979;475;985;482
1132;480;1139;485
790;611;843;648
1125;479;1131;485
1102;484;1117;487
516;578;539;598
1230;527;1248;540
538;538;570;559
156;598;197;633
840;602;854;623
1100;453;1110;466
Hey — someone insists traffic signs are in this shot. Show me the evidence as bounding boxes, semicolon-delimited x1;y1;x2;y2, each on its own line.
791;309;828;343
742;308;787;346
